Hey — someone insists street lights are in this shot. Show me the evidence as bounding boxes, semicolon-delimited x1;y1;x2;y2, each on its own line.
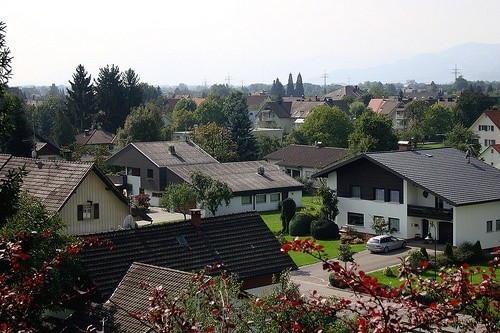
423;222;437;281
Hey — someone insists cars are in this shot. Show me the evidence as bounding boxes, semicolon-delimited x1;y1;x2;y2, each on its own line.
366;234;407;254
